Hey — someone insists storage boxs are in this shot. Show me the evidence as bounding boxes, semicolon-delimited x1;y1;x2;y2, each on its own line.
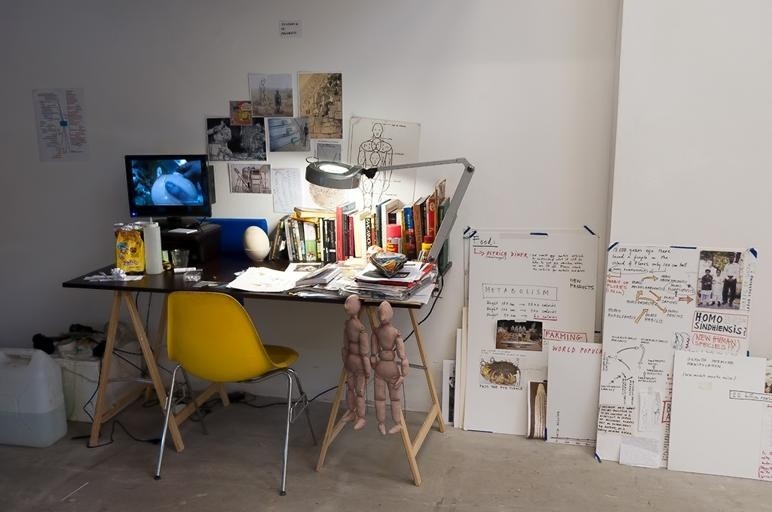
51;340;146;424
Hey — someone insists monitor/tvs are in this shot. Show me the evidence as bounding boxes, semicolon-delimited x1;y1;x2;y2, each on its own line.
124;154;212;230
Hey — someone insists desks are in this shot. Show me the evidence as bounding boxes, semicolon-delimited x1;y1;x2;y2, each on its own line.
61;255;445;488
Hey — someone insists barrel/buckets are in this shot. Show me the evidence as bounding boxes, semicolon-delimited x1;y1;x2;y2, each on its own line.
0;346;68;447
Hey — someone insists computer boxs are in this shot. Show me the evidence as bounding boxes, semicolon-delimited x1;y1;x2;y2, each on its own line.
141;223;222;264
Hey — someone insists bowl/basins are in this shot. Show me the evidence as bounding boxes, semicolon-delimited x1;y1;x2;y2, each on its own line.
243;246;272;263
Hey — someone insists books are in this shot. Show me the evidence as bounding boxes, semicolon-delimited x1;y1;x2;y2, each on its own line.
271;179;450;305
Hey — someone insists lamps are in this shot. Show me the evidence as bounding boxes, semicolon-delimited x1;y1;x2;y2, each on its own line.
304;152;477;262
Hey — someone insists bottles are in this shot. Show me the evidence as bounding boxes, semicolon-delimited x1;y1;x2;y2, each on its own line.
384;224;401;254
403;229;416;259
143;217;164;275
421;236;434;259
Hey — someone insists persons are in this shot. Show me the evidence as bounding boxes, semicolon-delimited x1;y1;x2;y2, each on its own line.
342;295;371;429
370;300;409;436
710;269;724;307
275;91;281;113
722;256;739;306
698;269;712;306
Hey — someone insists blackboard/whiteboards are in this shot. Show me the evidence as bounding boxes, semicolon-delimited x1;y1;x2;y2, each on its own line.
460;229;599;439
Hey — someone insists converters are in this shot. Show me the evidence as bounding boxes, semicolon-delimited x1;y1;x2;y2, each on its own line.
227;391;246;403
92;340;106;357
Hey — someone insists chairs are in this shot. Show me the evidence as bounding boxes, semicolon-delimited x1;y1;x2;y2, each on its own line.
151;291;318;496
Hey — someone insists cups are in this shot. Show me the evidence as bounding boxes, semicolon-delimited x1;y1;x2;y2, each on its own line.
171;248;190;268
